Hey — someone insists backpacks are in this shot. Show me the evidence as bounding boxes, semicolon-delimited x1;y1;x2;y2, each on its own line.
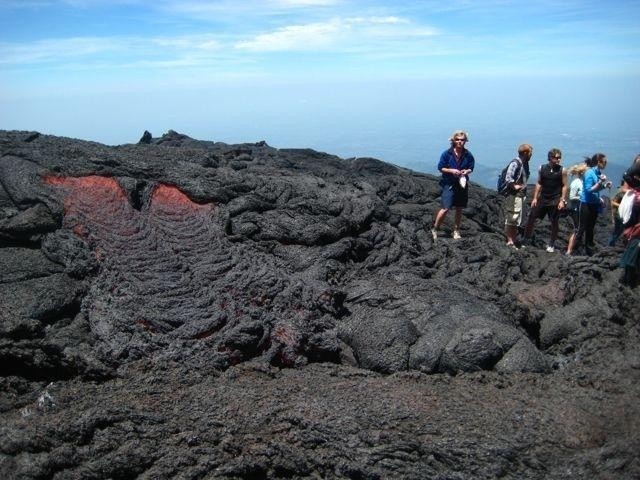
496;159;522;197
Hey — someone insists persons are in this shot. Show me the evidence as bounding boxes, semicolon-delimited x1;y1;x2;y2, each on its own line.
567;153;640;289
504;143;533;251
519;148;568;253
431;131;475;241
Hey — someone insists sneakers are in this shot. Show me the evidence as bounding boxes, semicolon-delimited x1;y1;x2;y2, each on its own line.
564;246;596;256
430;227;439;242
546;242;557;253
450;230;462;240
505;237;534;249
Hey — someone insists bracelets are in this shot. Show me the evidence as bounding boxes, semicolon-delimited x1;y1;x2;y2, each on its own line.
560;198;566;203
